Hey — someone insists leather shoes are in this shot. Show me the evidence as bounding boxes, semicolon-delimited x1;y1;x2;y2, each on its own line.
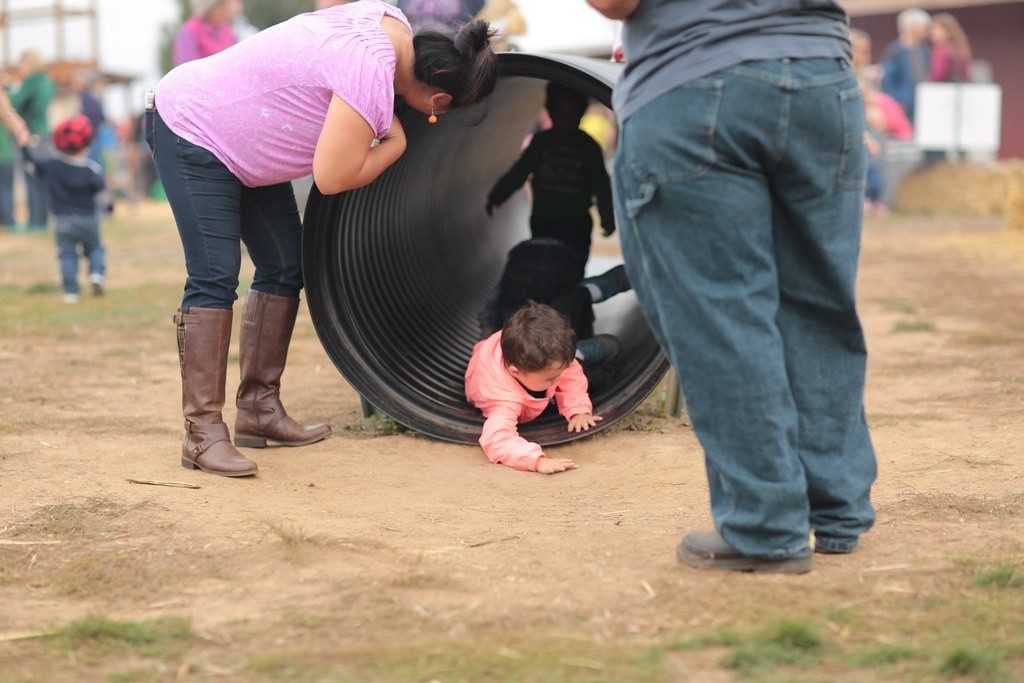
681;532;814;577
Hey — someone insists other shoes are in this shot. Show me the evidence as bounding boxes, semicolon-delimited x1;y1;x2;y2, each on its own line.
88;272;105;299
63;289;83;304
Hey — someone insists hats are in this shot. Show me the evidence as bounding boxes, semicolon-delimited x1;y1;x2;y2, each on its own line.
52;114;94;151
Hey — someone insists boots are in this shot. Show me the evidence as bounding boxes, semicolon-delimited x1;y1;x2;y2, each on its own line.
172;305;260;477
233;289;333;448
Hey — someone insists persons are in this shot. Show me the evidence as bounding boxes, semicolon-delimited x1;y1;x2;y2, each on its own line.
485;80;615;267
145;0;498;477
847;5;976;213
463;265;633;474
478;237;595;344
0;0;525;236
19;114;115;304
585;1;879;574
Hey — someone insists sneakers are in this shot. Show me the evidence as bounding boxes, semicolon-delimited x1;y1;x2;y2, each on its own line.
575;334;624;365
579;264;635;302
